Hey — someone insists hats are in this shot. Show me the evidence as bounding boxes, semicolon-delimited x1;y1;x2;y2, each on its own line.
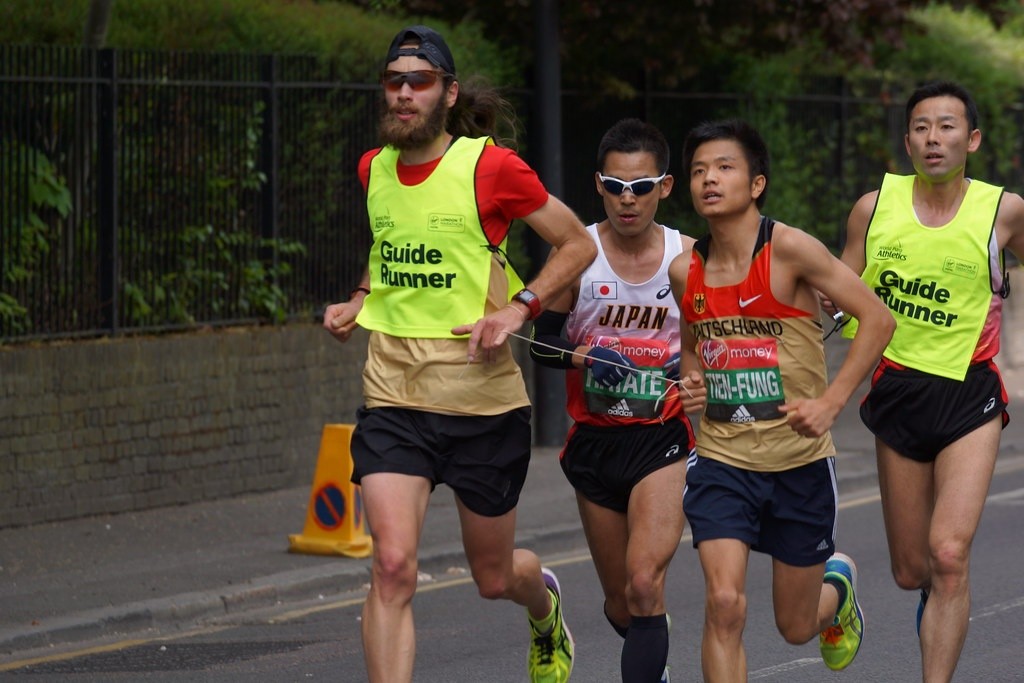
385;25;456;75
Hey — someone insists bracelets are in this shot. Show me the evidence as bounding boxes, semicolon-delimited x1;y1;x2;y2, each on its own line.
350;287;370;296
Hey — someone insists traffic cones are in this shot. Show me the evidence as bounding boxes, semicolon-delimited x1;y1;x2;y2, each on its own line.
289;424;372;558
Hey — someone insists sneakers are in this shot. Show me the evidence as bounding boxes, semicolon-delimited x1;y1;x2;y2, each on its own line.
820;552;865;672
526;567;575;683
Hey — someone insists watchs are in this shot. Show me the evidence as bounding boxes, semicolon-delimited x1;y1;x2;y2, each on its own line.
511;288;541;320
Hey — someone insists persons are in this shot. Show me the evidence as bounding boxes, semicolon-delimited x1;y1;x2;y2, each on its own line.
668;121;896;683
531;120;696;683
817;83;1024;683
324;27;598;683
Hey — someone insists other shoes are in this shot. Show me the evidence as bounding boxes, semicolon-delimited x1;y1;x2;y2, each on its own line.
659;612;672;683
916;586;931;637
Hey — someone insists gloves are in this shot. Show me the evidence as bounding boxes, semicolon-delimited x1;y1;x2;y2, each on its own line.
661;352;680;387
585;346;639;388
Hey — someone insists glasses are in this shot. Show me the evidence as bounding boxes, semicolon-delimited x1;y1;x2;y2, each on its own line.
599;172;667;196
381;69;437;92
